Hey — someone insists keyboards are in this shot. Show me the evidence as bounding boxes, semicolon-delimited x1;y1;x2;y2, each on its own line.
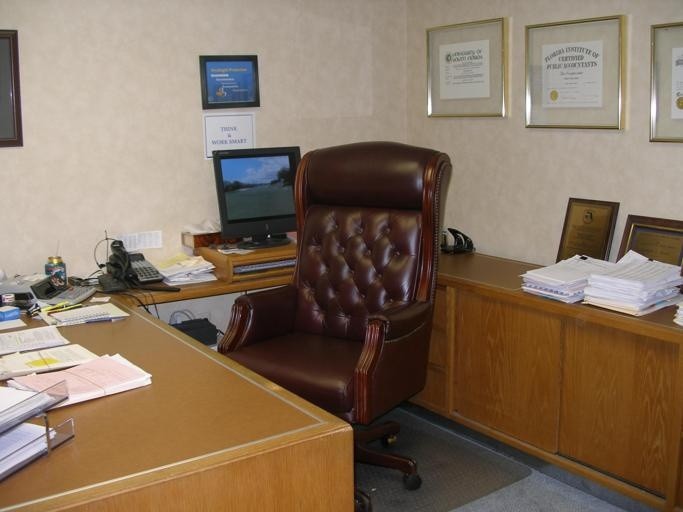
233;259;297;274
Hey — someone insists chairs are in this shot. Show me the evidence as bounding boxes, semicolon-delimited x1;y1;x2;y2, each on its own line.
216;141;453;512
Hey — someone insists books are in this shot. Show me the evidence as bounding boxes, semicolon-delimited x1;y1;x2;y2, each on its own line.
48;305;109;323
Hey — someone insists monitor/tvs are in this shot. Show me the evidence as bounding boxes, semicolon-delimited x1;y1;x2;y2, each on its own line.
212;145;303;250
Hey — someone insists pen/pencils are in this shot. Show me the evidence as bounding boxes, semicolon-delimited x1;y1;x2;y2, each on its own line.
85;317;124;324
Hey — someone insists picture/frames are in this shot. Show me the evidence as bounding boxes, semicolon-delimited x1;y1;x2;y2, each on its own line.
0;30;24;147
523;14;627;131
199;54;260;109
649;20;683;143
424;16;509;117
615;213;683;293
555;196;620;264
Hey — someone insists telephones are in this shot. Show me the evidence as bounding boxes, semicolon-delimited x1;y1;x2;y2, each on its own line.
109;253;164;282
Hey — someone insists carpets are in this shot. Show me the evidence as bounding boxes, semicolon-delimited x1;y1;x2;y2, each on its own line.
300;385;532;512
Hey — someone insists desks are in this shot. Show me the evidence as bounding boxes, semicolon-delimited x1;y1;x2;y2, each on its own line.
90;234;297;306
0;291;354;512
